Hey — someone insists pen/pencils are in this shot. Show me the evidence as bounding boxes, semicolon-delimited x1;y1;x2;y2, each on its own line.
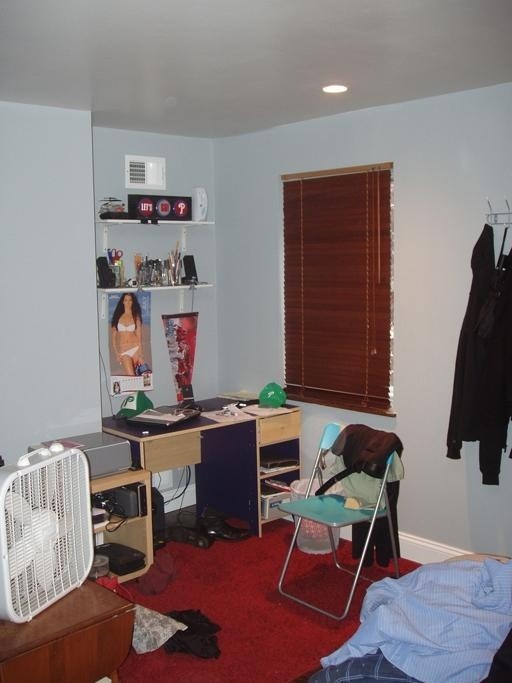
135;241;182;287
107;249;123;267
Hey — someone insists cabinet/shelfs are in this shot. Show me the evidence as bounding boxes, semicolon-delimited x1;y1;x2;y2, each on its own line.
97;219;215;293
89;468;154;585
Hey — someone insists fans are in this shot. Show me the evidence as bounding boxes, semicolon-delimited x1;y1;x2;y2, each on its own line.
0;444;94;624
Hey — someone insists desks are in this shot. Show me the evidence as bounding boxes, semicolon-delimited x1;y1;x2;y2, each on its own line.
0;580;135;683
102;397;303;538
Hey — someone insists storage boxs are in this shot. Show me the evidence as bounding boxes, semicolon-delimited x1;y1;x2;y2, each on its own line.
261;484;292;520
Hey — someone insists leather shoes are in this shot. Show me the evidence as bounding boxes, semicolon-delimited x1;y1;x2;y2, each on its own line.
197;515;250;541
170;525;213;549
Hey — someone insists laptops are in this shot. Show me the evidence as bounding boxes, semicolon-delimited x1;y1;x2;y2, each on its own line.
127;405;201;426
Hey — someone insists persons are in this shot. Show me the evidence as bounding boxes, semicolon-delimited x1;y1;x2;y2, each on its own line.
113;381;121;395
111;293;144;376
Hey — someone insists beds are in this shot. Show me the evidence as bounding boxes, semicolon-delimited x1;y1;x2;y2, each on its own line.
308;554;512;683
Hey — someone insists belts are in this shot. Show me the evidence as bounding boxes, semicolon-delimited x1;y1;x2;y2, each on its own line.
315;463;385;496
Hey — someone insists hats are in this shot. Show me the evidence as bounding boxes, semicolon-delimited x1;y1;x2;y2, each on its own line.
114;390;154;418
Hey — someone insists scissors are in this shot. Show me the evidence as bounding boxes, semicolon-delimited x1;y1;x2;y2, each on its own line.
111;249;123;266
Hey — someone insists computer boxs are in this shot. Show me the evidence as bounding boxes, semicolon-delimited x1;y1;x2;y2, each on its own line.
150;487;167;550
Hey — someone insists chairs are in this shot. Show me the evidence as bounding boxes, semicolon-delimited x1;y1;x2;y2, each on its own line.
278;422;400;621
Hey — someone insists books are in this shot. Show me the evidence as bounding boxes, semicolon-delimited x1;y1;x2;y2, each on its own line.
215;388;261;402
